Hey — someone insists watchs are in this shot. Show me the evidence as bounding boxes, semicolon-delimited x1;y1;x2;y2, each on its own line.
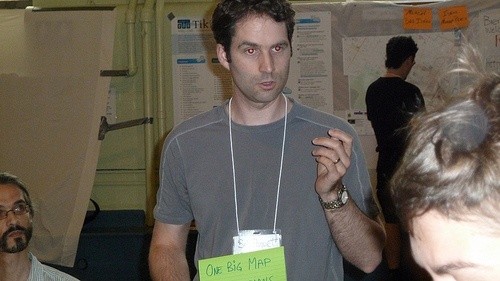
316;183;351;210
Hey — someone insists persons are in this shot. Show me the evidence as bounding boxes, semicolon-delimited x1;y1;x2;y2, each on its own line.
0;171;82;281
365;36;432;280
388;28;500;281
147;0;388;281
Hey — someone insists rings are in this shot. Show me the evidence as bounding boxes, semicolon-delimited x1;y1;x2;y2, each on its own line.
334;156;342;166
